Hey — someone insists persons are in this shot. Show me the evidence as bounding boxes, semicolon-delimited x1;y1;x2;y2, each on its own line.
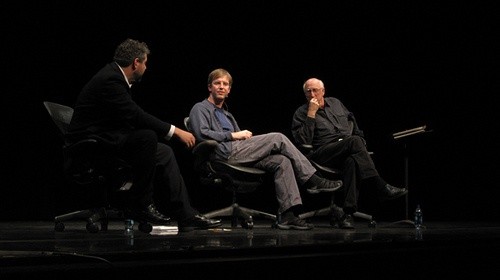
65;39;222;232
189;68;343;230
291;77;408;229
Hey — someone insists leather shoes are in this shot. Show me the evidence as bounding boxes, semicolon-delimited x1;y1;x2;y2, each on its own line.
138;203;172;225
177;212;225;232
375;183;408;200
339;211;355;228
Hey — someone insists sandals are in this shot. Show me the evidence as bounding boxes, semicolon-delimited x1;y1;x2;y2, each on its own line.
274;214;314;229
307;178;343;193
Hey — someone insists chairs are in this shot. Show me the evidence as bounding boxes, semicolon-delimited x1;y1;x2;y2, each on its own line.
298;111;378;228
43;101;153;237
184;110;277;230
